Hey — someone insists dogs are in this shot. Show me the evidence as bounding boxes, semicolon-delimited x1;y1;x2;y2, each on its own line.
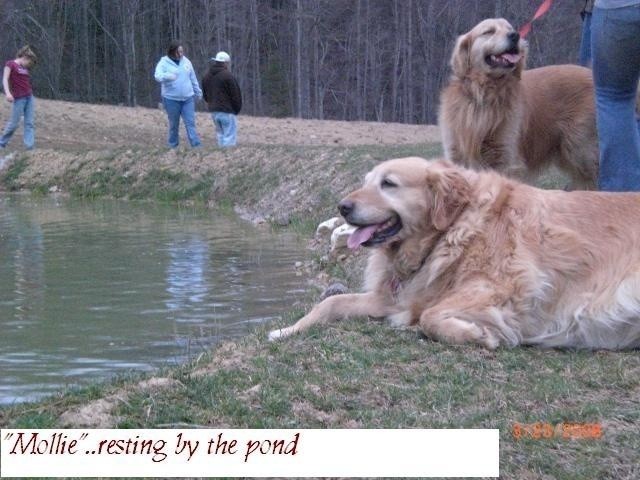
268;155;640;351
435;17;640;191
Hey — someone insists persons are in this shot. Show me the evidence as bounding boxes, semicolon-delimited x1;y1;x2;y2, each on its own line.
154;41;203;149
0;45;38;152
589;0;640;193
200;49;242;148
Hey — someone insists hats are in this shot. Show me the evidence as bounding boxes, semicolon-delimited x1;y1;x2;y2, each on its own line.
211;51;231;62
